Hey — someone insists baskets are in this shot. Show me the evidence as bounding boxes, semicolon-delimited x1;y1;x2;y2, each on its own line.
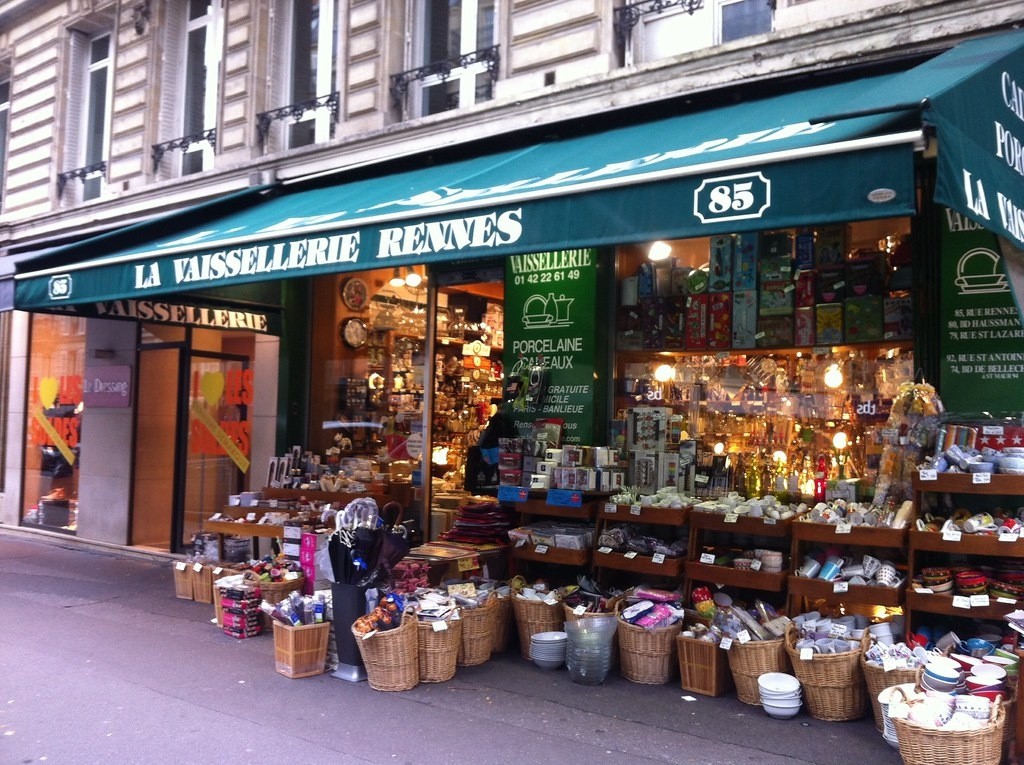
351;574;1017;764
243;576;305;631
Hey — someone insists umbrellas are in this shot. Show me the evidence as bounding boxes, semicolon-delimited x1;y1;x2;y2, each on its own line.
321;497;411;587
260;590;327;626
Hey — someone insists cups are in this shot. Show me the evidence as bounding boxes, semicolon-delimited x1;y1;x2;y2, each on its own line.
496;435;627;493
791;550;905;669
638;485;914;530
917;493;1024;537
467;577;561;606
907;621;1016;656
922;444;970;473
691;586;717;622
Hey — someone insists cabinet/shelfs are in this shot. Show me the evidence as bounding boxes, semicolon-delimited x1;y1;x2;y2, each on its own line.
384;329;503;417
201;482;416;560
904;469;1024;647
592;500;692;610
505;495;604;579
787;510;910;621
685;510;808;629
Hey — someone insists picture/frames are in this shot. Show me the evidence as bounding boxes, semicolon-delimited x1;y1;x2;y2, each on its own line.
342;277;369;311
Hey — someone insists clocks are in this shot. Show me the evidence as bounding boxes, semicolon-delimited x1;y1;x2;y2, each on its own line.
344;320;365;347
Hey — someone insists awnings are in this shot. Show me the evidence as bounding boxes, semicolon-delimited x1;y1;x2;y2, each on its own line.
12;29;1024;318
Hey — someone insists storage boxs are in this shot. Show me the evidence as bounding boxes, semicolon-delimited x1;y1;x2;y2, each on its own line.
330;582;371;684
659;452;679;493
37;500;70;527
507;519;596;550
339;377;369;412
39;445;74;478
824;477;862;504
615;224;919;351
679;440;697;493
627;407;674;452
239;491;262;508
628;451;658;495
497;436;629;490
282;521;323;561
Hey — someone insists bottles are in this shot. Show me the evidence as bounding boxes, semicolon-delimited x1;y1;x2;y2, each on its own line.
611;345;913;509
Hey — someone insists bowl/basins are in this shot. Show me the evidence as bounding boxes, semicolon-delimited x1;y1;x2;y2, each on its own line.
757;672;803;719
877;656;1019;749
970;446;1024;475
732;548;783;573
529;629;567;670
564;615;617;686
912;561;1024;603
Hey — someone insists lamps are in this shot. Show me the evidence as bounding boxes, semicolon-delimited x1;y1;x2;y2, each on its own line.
405;266;422;288
388;267;405;287
